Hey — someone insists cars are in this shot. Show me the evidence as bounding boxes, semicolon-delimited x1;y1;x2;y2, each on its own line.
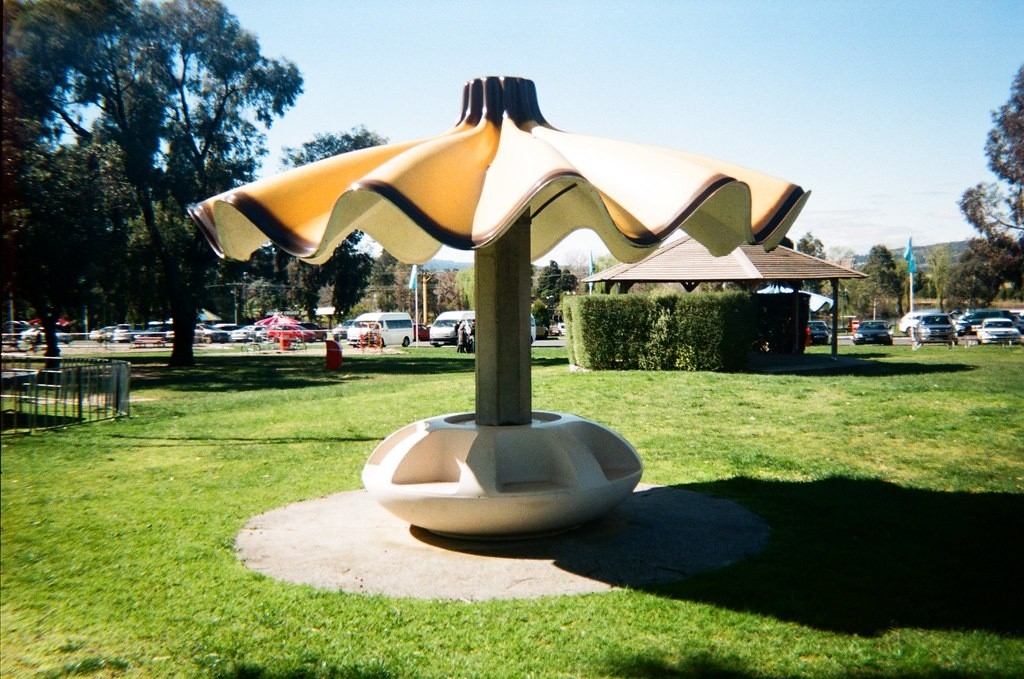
89;321;327;343
852;319;893;345
413;324;430;340
21;326;73;344
332;320;368;337
806;319;830;345
897;307;1024;344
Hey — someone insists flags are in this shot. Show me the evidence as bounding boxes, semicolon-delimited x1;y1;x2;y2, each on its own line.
904;237;917;273
590;255;595;289
408;265;418;289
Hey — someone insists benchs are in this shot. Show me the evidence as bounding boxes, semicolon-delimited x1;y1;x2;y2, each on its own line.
133;337;167;347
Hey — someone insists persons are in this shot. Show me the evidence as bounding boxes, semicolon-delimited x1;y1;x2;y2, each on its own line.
455;319;475;353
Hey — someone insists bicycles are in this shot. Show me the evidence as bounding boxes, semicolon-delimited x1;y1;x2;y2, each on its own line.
240;331;261;352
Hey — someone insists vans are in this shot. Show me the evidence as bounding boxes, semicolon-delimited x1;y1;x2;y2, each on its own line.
429;307;549;345
347;312;414;347
